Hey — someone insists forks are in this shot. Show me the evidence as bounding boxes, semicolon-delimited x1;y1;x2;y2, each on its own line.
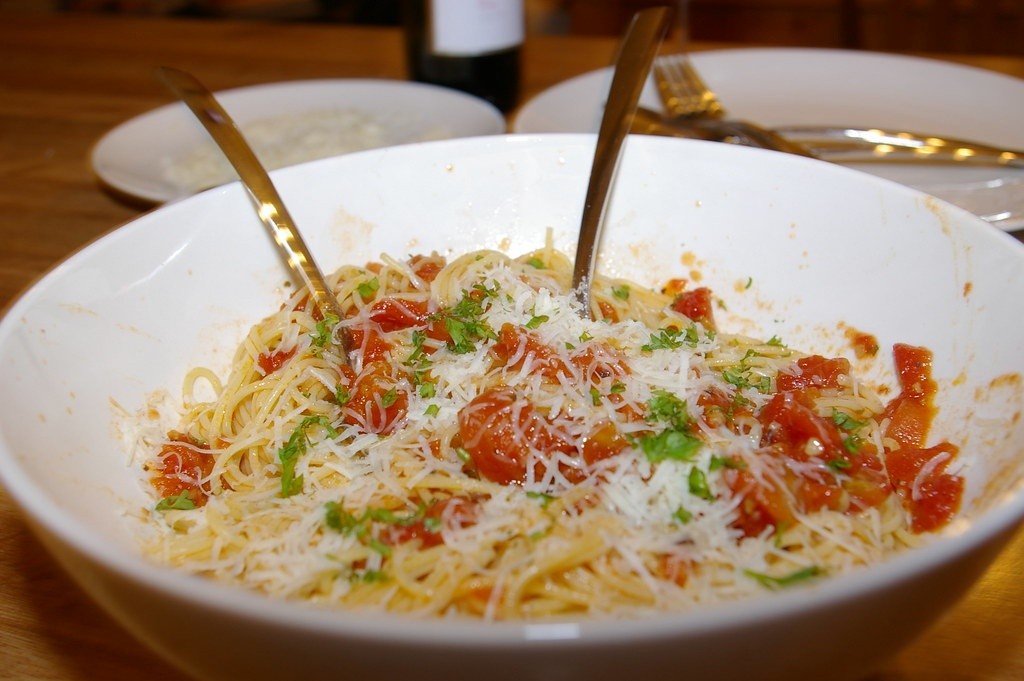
652;57;820;158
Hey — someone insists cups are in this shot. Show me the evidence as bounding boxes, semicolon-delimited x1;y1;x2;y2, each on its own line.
406;0;522;116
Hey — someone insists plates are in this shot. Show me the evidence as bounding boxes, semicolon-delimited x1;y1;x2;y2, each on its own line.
90;78;507;204
0;133;1024;681
513;48;1024;232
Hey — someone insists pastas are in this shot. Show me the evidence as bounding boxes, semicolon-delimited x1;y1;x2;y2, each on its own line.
145;245;970;621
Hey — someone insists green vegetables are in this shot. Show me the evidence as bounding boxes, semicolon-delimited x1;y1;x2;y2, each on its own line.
155;257;865;589
